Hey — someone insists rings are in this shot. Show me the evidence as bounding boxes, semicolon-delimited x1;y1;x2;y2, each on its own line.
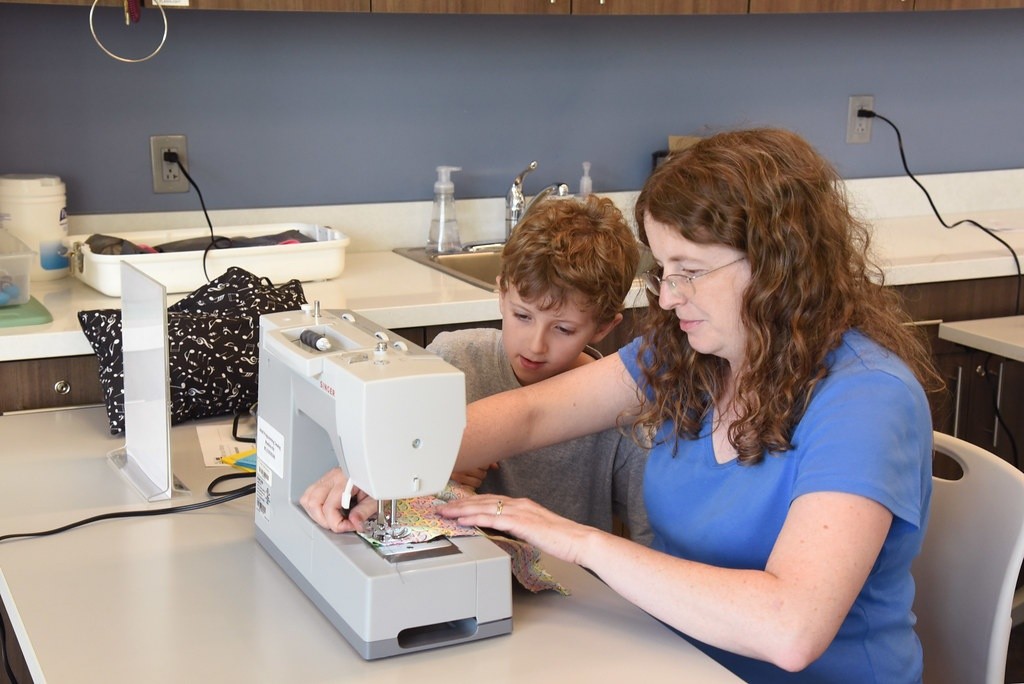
496;502;503;515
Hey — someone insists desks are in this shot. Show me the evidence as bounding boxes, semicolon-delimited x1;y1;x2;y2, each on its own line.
936;316;1024;460
2;394;752;681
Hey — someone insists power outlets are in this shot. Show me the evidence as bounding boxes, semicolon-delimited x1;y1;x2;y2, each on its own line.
845;96;874;143
148;136;192;195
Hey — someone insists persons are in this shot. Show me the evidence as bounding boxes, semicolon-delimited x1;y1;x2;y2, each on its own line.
425;195;652;548
299;129;941;684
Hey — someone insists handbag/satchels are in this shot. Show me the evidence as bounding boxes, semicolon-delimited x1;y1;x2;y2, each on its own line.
76;267;308;436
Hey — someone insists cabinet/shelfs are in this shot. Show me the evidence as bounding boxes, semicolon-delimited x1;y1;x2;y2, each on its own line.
3;277;1023;598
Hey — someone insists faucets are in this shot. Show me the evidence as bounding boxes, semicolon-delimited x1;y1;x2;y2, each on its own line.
504;160;569;245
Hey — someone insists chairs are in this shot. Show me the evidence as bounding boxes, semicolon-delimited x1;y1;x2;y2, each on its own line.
907;429;1024;684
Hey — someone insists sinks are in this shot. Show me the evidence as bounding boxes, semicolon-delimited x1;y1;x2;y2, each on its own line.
431;249;658;291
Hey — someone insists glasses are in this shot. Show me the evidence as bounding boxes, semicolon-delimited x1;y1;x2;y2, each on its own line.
641;255;747;298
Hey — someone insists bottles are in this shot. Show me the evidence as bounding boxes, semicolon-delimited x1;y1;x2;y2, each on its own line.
1;173;71;282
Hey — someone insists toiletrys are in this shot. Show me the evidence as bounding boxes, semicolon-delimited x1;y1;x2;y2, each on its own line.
579;161;593;204
424;165;464;256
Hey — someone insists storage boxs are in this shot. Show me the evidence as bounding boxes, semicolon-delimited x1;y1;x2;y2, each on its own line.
59;222;353;295
